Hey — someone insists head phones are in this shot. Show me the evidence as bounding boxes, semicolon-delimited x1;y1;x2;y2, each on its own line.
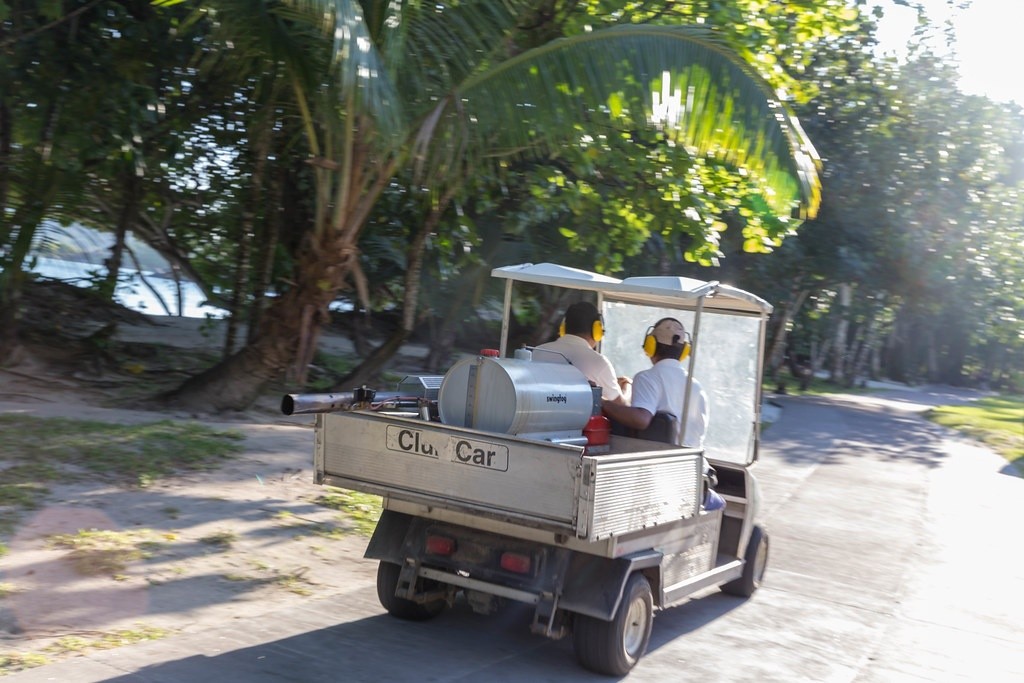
642;317;692;362
559;312;605;341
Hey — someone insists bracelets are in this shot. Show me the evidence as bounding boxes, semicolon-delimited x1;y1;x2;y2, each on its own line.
619;378;632;385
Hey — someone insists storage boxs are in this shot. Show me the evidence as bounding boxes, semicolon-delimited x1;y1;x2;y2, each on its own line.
584;415;611;457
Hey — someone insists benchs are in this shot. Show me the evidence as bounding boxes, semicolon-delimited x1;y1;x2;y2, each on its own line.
606;409;678;444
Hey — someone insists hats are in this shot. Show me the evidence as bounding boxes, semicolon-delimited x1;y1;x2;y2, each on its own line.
652;316;686;347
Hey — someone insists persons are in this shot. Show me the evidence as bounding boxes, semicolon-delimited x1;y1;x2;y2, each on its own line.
603;321;727;509
533;304;633;408
